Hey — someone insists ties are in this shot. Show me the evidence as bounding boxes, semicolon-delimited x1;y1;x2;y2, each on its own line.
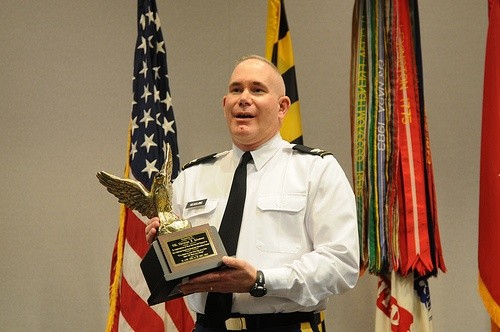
204;151;254;322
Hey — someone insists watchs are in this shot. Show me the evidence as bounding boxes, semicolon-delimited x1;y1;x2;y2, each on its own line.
251;270;267;298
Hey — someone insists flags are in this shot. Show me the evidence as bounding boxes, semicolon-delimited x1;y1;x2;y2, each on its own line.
107;0;197;332
266;0;325;332
350;0;447;332
478;0;500;332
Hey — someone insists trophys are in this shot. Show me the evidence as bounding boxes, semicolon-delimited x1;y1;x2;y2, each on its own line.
97;142;230;307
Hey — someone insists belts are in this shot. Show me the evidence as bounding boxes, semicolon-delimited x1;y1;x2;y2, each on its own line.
196;314;313;330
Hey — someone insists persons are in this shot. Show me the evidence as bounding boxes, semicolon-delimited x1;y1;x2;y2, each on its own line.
144;56;360;332
